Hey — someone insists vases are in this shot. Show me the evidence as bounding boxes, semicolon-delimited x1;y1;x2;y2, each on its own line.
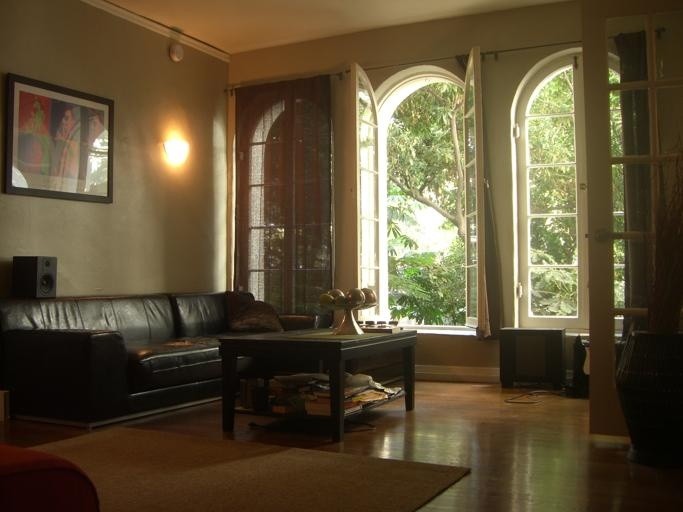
615;327;682;470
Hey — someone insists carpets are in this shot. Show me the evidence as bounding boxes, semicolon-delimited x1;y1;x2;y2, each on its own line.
0;420;472;512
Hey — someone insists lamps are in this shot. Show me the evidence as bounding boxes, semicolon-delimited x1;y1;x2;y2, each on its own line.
163;135;190;168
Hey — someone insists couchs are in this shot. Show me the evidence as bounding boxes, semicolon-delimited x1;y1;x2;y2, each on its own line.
0;290;298;434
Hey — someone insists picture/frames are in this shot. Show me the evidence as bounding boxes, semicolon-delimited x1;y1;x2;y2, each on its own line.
3;72;115;204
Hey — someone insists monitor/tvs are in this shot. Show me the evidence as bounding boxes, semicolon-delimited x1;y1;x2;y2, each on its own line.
499;328;563;390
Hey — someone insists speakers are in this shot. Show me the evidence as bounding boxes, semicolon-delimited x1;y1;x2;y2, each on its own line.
12;256;57;298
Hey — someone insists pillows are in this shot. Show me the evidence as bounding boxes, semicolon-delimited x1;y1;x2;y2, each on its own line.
223;291;285;334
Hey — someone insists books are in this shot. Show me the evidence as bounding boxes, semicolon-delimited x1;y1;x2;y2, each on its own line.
267;367;389;417
